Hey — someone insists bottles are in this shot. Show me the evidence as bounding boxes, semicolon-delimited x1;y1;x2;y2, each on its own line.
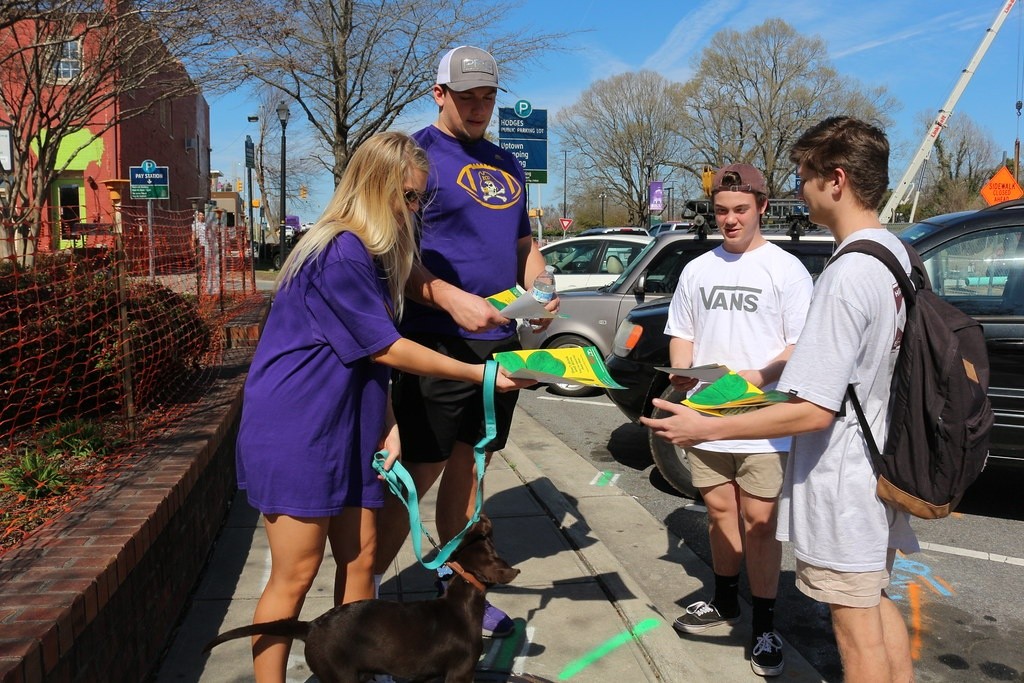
523;265;555;329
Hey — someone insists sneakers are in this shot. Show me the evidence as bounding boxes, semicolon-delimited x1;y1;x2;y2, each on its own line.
674;596;742;633
751;622;785;676
482;599;516;637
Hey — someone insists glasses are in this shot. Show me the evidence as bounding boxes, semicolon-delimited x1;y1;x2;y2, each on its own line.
795;176;803;190
402;190;420;206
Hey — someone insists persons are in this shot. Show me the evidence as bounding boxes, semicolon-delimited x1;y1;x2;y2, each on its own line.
639;114;922;683
663;164;814;675
192;209;206;279
237;126;538;683
334;46;560;608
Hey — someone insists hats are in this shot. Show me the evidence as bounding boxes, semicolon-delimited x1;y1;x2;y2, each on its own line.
438;46;510;94
712;164;768;196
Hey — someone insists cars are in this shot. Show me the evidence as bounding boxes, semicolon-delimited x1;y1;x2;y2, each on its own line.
301;222;314;230
279;226;294;237
515;221;694;326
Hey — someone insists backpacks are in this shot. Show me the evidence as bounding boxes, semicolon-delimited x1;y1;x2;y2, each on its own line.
822;238;992;522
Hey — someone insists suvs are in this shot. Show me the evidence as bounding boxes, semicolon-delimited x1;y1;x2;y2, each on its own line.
513;214;939;398
599;200;1024;513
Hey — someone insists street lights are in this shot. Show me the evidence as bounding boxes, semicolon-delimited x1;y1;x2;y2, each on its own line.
275;99;291;263
561;150;571;219
246;113;266;257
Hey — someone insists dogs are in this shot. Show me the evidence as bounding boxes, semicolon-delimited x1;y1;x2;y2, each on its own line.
198;513;520;683
